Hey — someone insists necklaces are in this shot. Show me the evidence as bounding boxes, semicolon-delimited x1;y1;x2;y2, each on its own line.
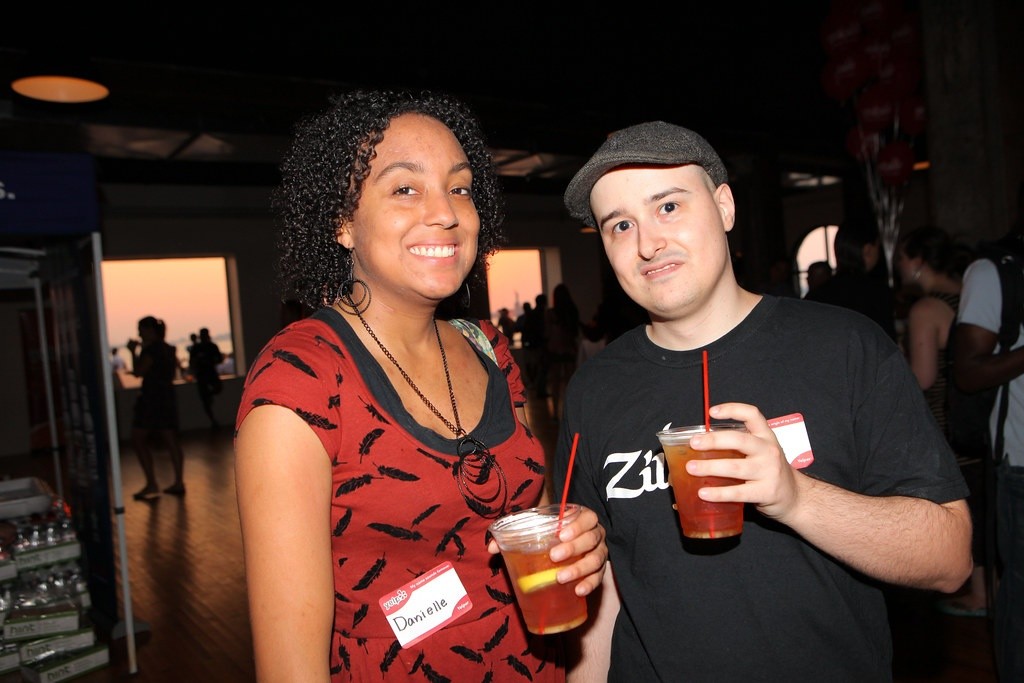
344;290;507;519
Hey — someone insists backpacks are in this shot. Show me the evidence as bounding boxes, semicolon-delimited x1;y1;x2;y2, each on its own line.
944;255;1024;467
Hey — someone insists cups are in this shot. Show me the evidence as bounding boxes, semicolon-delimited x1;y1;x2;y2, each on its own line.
487;502;589;636
655;422;746;538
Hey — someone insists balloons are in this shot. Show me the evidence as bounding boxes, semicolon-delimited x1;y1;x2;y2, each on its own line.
824;0;926;183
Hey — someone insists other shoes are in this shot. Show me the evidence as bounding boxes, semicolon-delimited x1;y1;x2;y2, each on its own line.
134;482;186;498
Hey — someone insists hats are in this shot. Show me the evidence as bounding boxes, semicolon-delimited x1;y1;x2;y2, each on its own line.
564;121;729;230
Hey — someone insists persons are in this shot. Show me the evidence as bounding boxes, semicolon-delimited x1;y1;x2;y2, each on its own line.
233;88;609;683
500;275;650;434
802;181;1023;683
109;316;225;499
554;120;976;683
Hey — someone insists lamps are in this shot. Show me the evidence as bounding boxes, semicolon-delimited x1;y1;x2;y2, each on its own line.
11;44;108;102
